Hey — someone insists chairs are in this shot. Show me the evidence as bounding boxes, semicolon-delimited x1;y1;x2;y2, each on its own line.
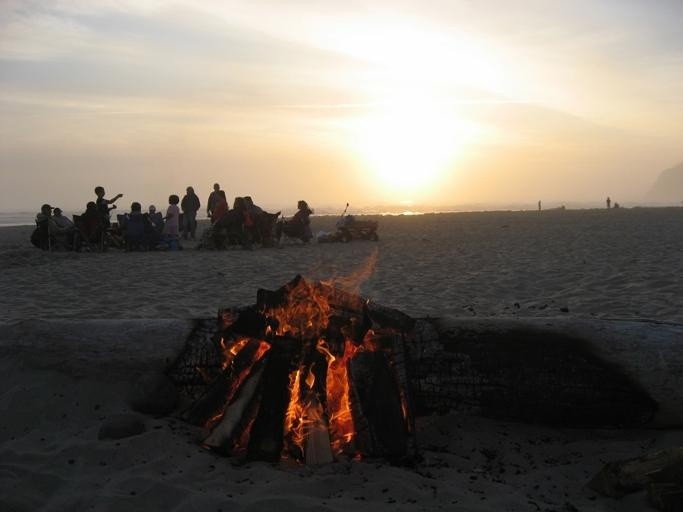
27;202;318;252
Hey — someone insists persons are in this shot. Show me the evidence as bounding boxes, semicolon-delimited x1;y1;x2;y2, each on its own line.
605;196;611;208
30;184;313;253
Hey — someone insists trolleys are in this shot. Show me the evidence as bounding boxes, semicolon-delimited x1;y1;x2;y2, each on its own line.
335;202;378;243
